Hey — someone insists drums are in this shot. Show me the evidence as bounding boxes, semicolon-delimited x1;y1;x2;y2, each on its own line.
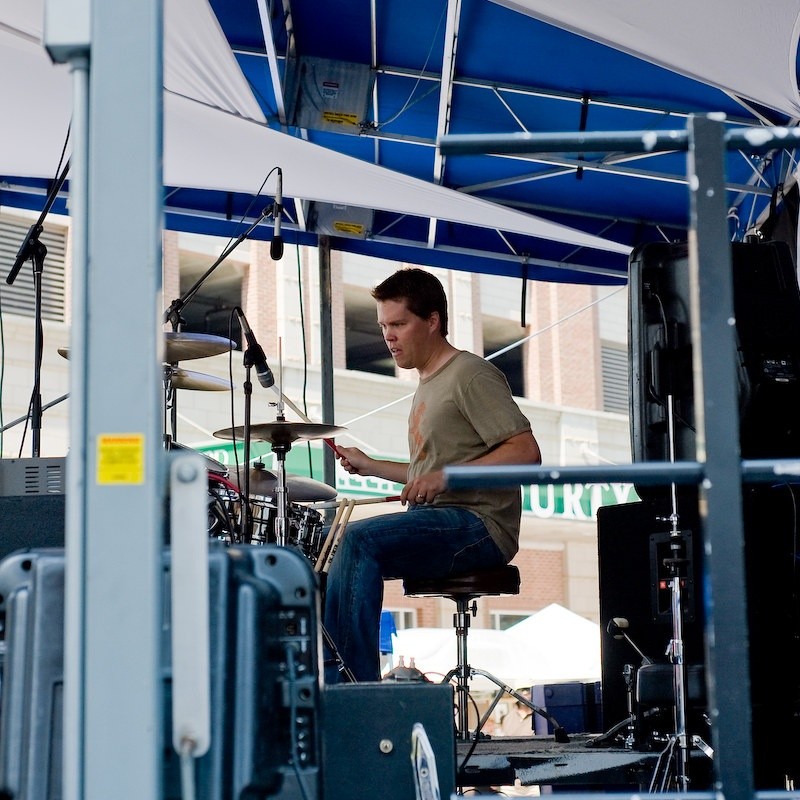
209;488;325;563
168;439;230;489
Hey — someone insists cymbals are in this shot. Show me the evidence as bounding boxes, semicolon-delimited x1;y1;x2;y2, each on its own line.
160;331;238;363
218;463;339;504
212;421;349;442
56;347;238;392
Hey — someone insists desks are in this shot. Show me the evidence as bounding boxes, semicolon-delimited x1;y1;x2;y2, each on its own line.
379;610;397;672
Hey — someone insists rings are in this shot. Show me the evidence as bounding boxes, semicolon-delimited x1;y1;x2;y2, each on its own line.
417;494;425;498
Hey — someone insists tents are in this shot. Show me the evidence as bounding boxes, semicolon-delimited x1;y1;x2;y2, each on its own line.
380;602;603;691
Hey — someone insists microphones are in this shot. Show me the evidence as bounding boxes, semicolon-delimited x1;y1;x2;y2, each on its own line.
237;308;275;388
271;170;283;260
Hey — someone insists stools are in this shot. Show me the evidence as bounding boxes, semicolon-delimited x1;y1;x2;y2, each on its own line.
403;565;571;743
637;665;715;793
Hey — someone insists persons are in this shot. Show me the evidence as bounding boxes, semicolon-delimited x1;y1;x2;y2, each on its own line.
321;268;542;697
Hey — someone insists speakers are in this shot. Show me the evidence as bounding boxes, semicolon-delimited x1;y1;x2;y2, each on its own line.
0;548;67;800
160;542;317;800
595;486;800;791
625;241;800;503
